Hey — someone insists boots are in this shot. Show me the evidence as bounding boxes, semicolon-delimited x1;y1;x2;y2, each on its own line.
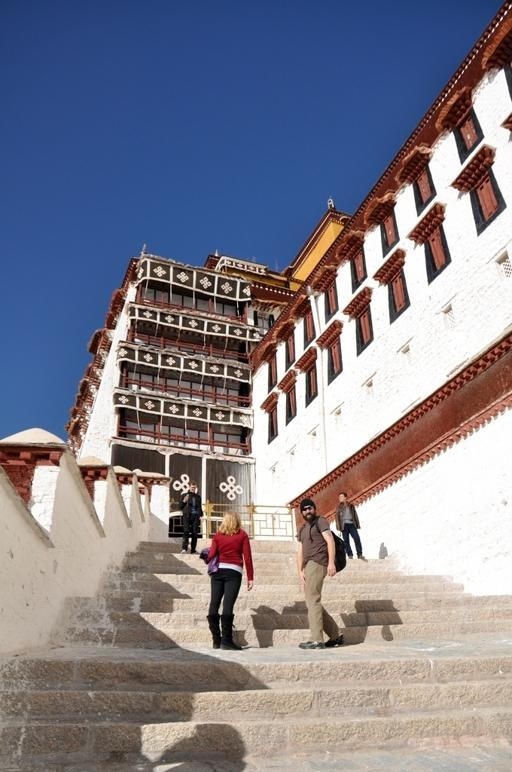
207;614;241;650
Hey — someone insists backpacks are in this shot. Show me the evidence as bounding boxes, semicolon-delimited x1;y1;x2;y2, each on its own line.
315;516;345;572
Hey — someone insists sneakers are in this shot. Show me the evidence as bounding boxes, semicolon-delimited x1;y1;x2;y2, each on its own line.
349;554;364;559
181;549;201;555
299;634;343;649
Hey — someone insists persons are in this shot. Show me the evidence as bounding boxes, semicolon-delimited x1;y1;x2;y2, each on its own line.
294;497;347;650
203;509;256;653
333;491;367;561
177;480;203;555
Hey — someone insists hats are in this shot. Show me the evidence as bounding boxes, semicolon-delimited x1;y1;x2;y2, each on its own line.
300;498;316;512
